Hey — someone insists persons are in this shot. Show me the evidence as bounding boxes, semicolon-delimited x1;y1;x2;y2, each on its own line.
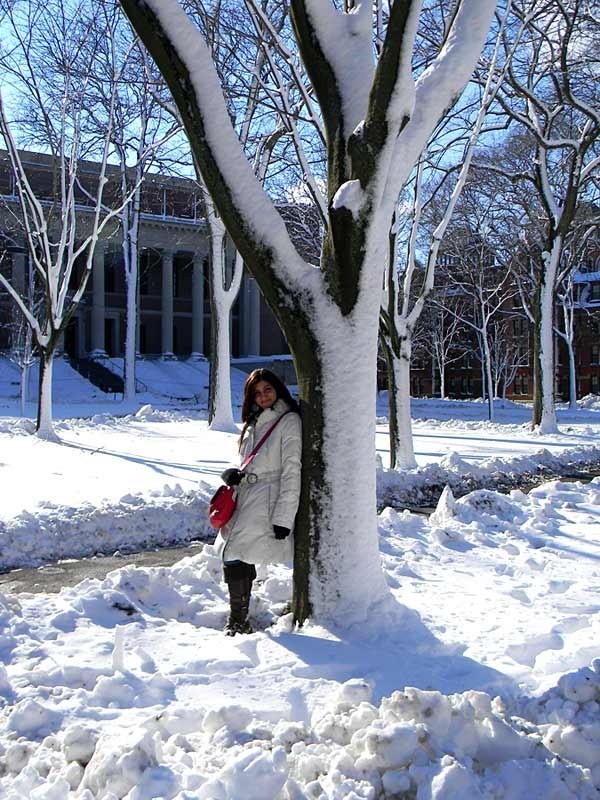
214;369;303;636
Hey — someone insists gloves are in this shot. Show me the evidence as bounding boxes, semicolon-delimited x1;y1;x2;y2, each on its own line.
272;525;290;540
222;467;245;486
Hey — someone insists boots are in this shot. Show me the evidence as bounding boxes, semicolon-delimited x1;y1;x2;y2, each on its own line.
226;564;254;635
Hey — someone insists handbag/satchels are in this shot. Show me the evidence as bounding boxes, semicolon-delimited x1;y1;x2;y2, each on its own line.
209;485;234;528
